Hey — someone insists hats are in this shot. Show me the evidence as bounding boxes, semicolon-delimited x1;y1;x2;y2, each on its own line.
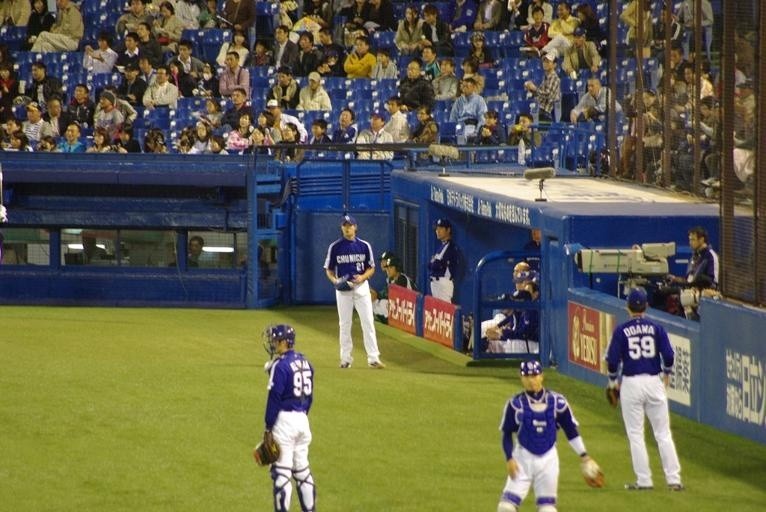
267;100;281;107
626;288;646;304
339;215;540;286
307;72;321;82
100;92;115;104
471;27;587;61
26;102;43;112
124;63;139;71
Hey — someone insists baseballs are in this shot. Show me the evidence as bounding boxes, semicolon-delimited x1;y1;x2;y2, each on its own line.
346;280;354;289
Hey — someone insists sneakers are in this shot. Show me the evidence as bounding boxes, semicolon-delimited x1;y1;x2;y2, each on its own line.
368;362;385;369
625;483;653;490
338;363;350;368
668;484;684;490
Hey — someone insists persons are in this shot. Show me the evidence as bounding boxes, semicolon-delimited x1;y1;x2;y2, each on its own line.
604;285;685;490
188;236;211;268
241;244;271;280
481;290;538;355
496;357;604;512
323;216;386;371
502;262;530;299
682;227;718;317
370;252;414;315
252;324;317;512
0;0;757;199
428;218;462;304
77;234;107;264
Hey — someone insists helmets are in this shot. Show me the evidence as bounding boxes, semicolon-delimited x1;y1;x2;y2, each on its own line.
261;325;294;360
520;360;541;376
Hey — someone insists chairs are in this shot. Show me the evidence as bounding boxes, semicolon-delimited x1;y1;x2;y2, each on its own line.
0;1;732;178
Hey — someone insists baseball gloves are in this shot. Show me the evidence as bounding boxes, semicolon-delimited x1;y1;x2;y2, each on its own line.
582;459;604;488
336;273;355;291
254;430;280;465
606;380;620;407
